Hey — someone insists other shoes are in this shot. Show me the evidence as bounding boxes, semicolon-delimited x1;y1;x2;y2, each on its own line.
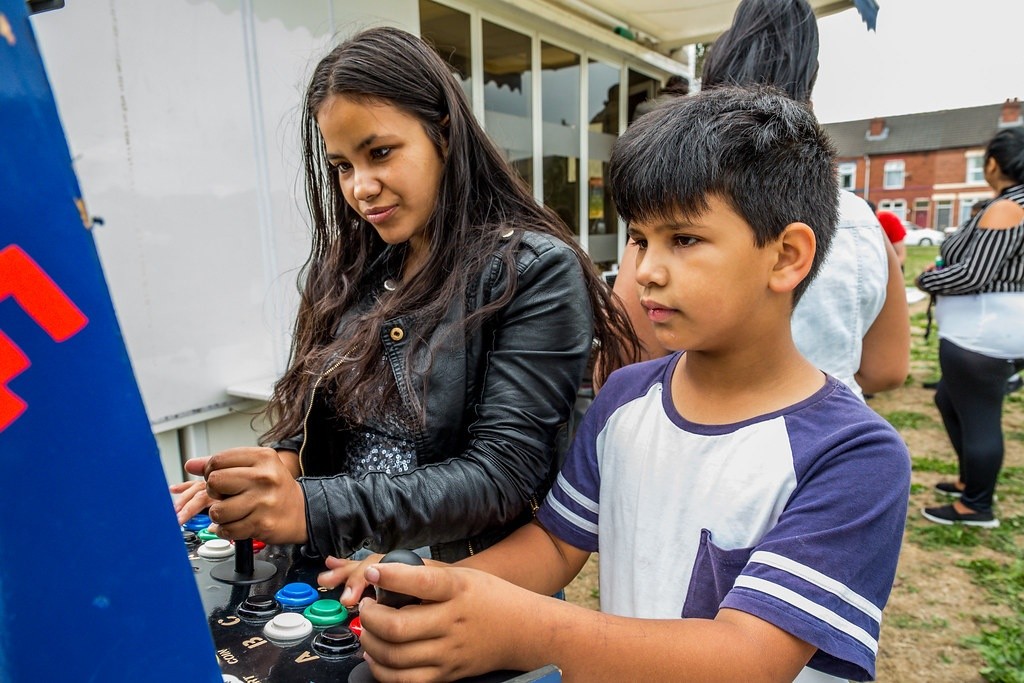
923;504;1001;528
923;379;940;389
1002;377;1024;393
935;482;998;501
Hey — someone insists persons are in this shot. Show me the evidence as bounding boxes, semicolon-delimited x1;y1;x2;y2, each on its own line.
317;86;911;683
167;26;651;603
914;126;1023;529
592;1;910;409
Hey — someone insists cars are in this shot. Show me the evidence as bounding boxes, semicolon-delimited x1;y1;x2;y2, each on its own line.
903;222;946;247
